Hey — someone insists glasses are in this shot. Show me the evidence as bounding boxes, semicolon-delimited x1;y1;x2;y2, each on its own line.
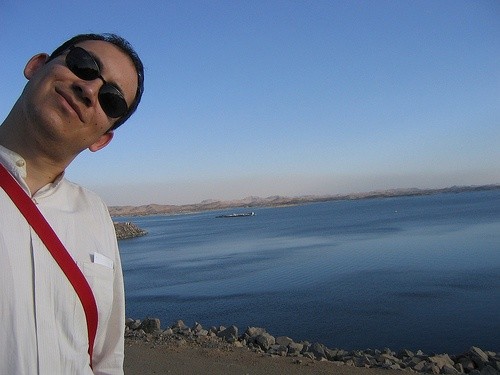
62;43;129;121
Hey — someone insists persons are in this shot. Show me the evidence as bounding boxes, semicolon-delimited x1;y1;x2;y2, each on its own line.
0;34;145;375
